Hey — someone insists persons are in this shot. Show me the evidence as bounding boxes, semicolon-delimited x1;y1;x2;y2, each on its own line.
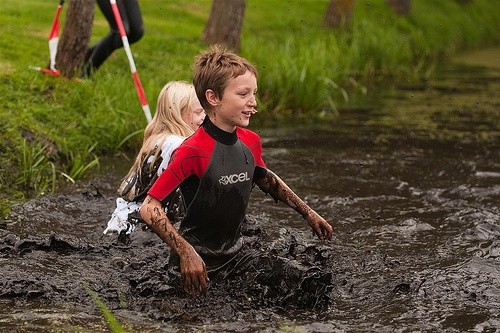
80;0;144;80
103;81;208;236
141;43;332;297
